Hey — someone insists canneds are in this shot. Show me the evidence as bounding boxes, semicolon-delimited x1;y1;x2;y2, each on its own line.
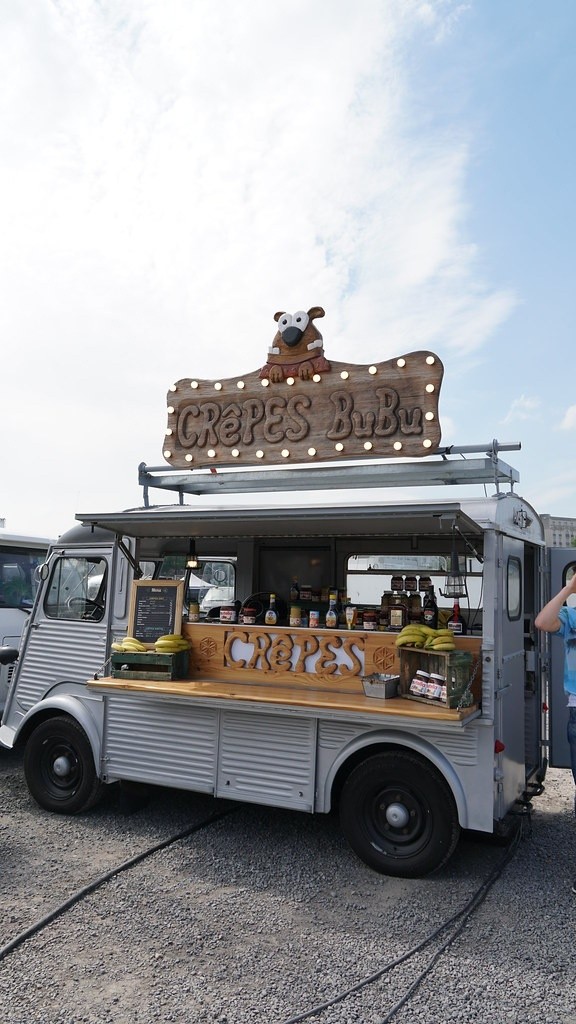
220;601;238;623
387;603;407;632
244;607;256;624
309;610;320;628
300;584;347;602
408;669;456;703
189;601;199;621
381;572;432;619
290;604;302;626
364;608;378;630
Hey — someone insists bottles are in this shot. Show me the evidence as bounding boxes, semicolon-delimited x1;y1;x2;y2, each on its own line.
188;602;200;622
446;598;467;635
325;594;340;629
263;593;279;626
289;577;300;605
423;586;439;629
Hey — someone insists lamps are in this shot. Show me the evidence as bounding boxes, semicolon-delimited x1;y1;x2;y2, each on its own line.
185;538;203;570
439;527;469;598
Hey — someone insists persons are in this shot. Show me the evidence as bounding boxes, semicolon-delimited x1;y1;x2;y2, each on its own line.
535;573;576;894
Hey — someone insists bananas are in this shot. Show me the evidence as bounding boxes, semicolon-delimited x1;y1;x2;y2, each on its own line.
396;623;456;649
111;634;190;653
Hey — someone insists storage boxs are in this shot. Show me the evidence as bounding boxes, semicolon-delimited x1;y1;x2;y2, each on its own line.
110;646;190;681
362;672;401;699
398;645;473;709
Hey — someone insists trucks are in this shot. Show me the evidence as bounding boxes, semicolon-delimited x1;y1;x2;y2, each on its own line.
0;440;576;876
